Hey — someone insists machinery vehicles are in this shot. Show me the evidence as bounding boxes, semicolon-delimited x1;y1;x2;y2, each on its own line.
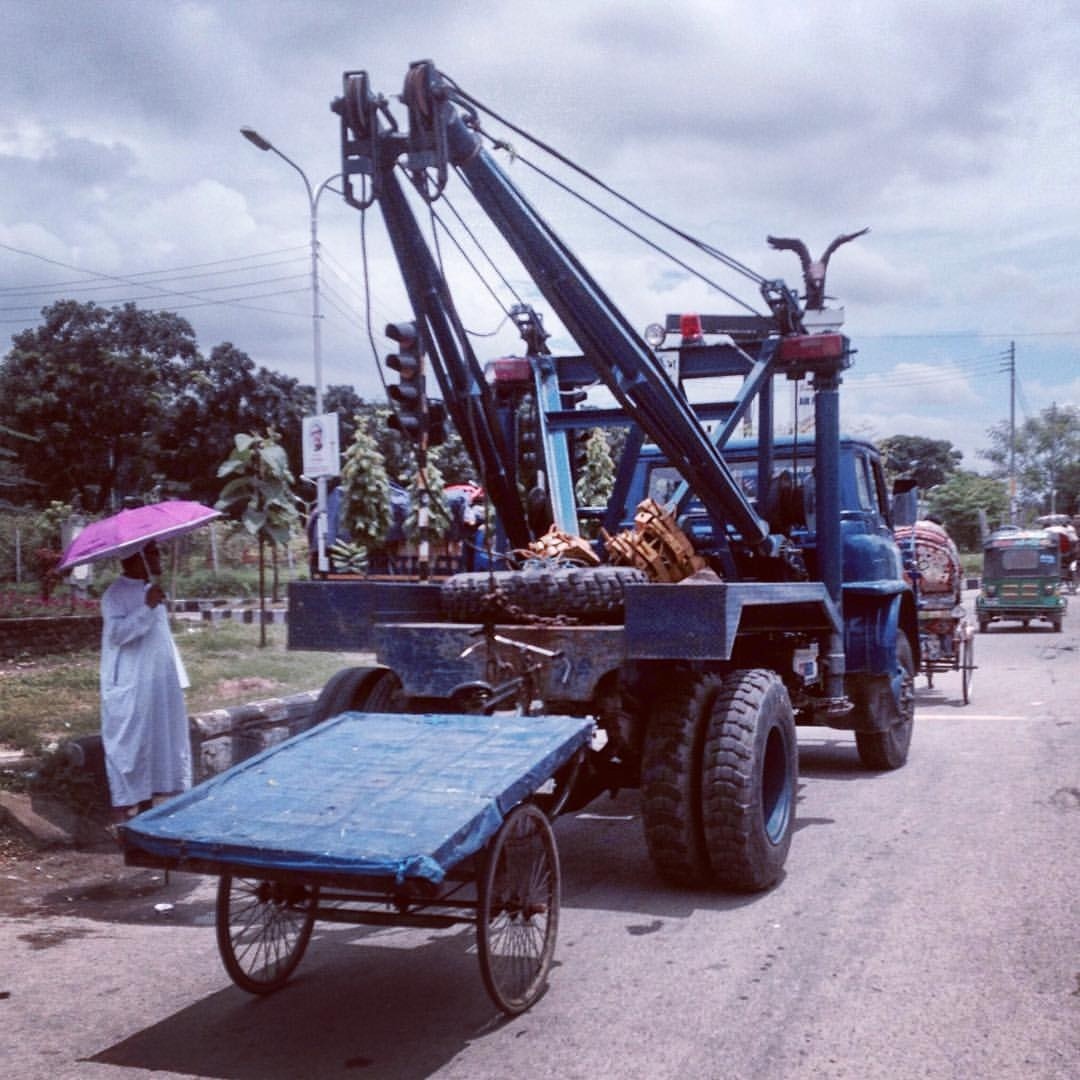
286;60;922;898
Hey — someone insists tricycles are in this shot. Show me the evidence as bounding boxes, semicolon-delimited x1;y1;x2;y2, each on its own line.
893;522;982;704
117;636;596;1016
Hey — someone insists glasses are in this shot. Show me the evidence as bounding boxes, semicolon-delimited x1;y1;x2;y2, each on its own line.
139;549;160;559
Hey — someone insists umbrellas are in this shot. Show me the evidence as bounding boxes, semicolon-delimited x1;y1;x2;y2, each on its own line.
60;502;221;583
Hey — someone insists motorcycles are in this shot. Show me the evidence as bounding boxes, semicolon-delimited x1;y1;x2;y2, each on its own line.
976;526;1068;634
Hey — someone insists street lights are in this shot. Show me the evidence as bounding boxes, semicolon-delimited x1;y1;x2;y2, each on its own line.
241;125;343;572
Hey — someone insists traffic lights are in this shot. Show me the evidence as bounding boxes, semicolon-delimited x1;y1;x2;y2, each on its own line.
519;392;591;484
427;397;449;448
386;320;422;441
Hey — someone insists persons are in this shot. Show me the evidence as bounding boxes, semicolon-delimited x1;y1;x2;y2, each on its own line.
101;541;190;851
920;513;1079;661
